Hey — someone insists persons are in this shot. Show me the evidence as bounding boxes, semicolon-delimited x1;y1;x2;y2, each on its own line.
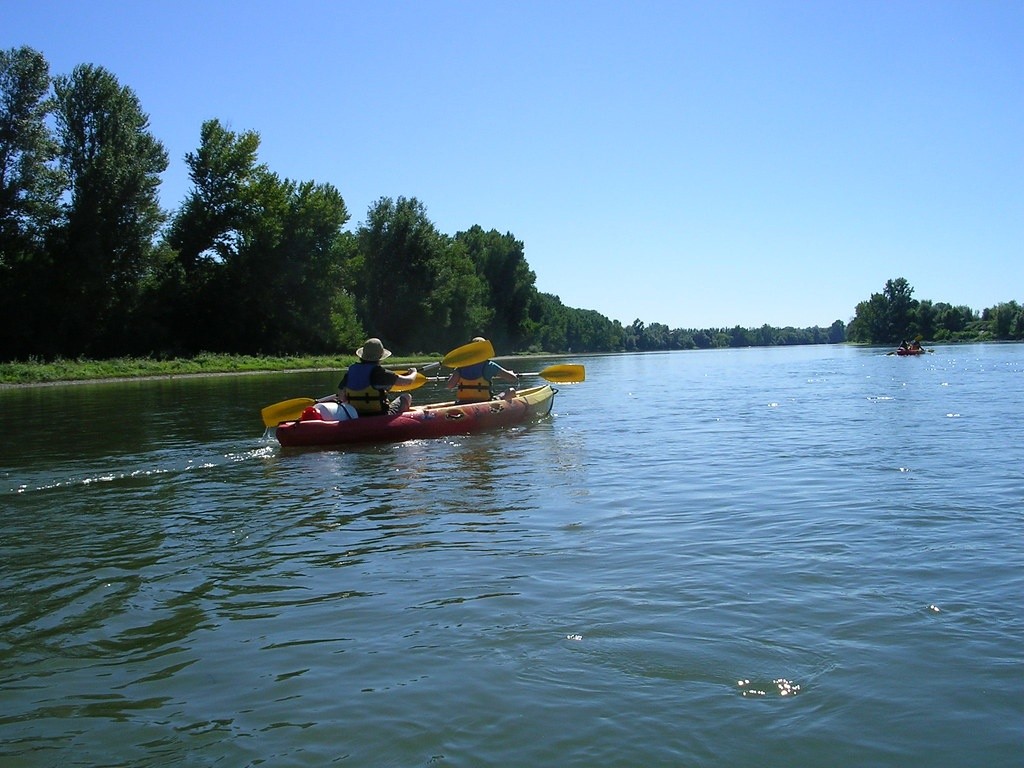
448;336;519;406
912;336;924;351
899;339;908;349
338;338;417;417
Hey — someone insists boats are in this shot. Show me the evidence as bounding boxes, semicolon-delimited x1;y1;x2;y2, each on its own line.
897;346;926;355
276;385;559;449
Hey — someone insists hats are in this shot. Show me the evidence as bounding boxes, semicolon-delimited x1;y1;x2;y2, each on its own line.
355;338;392;361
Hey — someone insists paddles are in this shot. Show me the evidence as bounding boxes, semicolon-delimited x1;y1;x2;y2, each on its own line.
262;340;499;429
387;363;586;391
887;334;923;355
915;347;934;352
